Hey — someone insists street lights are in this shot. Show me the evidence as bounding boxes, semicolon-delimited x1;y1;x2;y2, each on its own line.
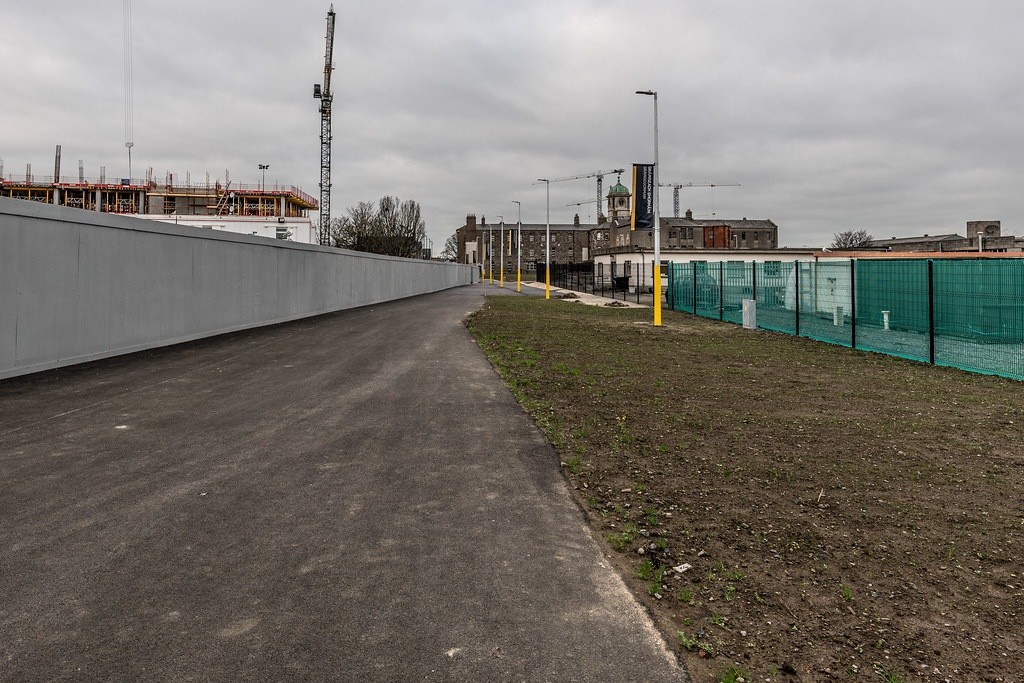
636;90;663;327
537;179;550;300
489;224;492;284
496;215;503;288
476;236;479;265
512;200;521;292
482;232;486;283
